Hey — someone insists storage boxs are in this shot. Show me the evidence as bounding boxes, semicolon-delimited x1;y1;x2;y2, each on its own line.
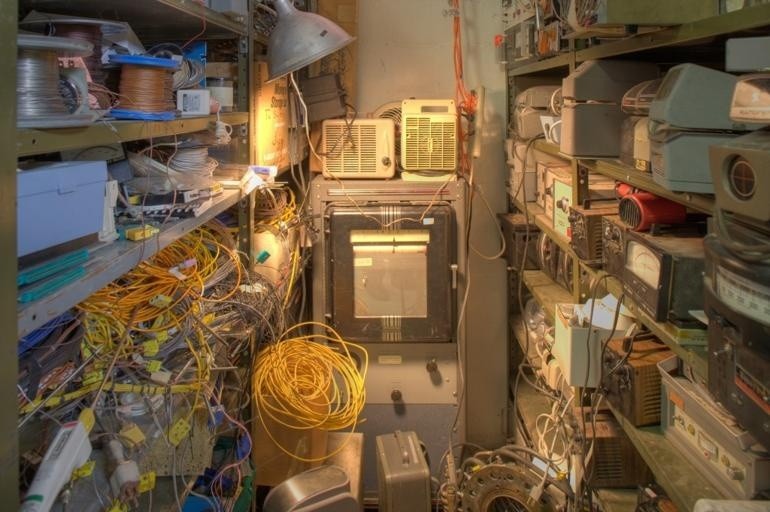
252;61;291;172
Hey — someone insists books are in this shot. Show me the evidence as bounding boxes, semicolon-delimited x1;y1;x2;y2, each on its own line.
140;196;215;219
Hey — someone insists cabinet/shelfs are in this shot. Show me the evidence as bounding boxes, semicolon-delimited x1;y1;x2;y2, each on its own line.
502;1;770;512
2;2;309;511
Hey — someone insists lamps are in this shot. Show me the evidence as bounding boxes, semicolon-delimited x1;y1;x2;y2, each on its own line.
265;0;358;85
